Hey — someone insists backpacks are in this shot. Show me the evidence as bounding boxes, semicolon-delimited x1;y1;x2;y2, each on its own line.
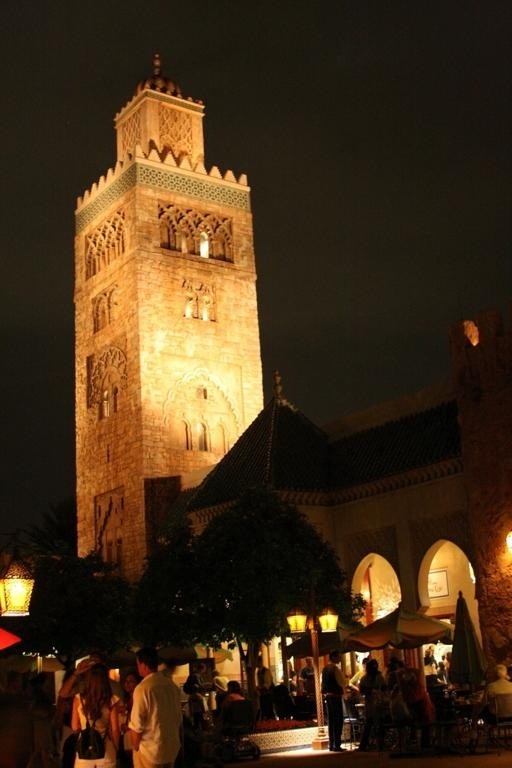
75;696;107;760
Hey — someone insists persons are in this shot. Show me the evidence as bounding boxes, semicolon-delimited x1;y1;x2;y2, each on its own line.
479;664;512;743
183;657;317;749
126;647;181;768
322;652;347;752
52;695;72;728
122;668;142;733
158;662;175;677
1;650;23;752
29;671;57;767
58;658;120;768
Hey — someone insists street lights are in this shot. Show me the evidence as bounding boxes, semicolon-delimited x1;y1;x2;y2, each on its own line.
285;601;341;738
1;549;38;618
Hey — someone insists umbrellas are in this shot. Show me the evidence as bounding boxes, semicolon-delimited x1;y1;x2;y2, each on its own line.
284;631;350;686
345;602;453;713
449;590;486;743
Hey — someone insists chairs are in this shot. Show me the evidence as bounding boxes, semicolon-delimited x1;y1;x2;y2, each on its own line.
342;689;512;757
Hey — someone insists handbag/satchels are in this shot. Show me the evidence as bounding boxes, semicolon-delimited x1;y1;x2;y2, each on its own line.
372;689;394;709
